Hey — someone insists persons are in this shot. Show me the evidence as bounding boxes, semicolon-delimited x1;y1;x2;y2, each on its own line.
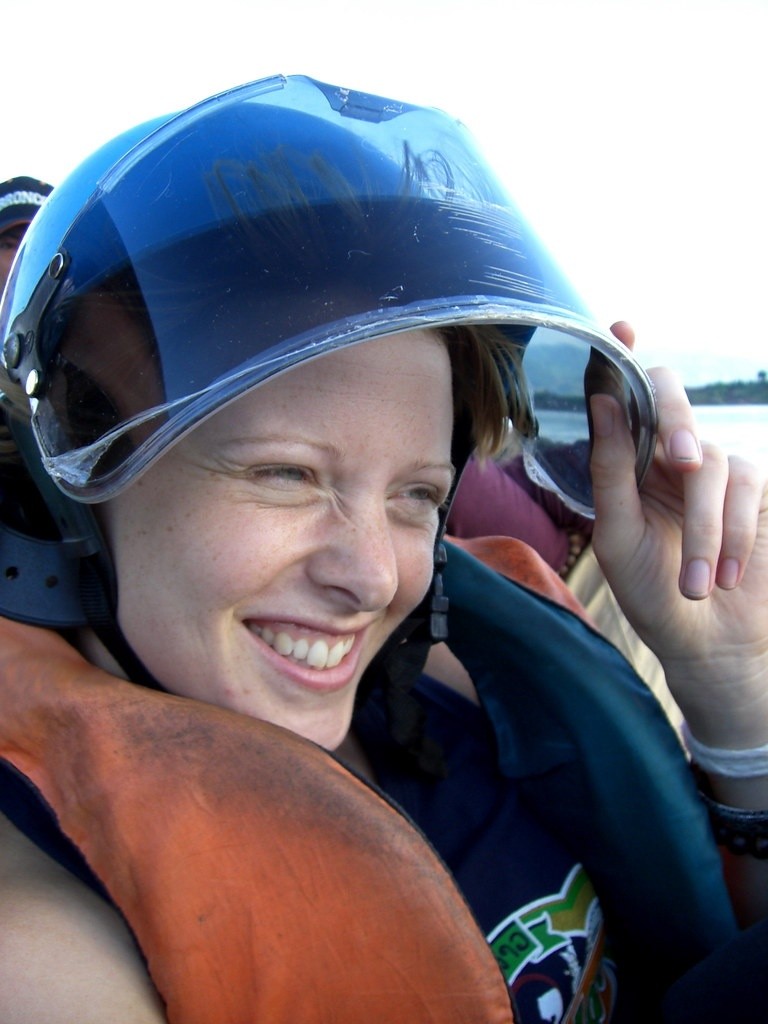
0;70;768;1024
0;176;54;464
446;432;689;764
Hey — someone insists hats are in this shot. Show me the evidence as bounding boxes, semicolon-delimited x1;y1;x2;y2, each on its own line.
0;175;57;235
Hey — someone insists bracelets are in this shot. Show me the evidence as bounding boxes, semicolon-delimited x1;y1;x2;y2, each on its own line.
559;528;580;579
678;723;768;777
695;785;768;862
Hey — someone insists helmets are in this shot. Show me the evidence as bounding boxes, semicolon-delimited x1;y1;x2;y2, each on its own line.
0;73;539;488
